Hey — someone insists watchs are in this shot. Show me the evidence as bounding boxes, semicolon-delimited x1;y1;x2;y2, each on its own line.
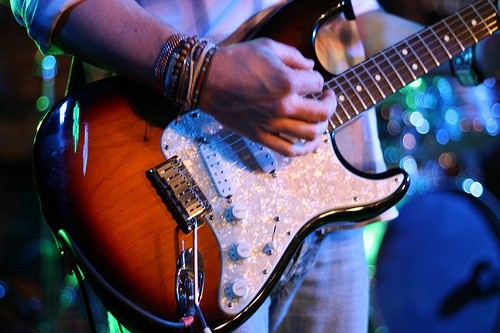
450;44;483;88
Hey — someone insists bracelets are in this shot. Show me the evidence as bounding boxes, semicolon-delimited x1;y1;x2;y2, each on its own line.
153;31;217;111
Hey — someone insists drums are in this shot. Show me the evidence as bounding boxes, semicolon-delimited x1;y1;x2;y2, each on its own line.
366;174;499;332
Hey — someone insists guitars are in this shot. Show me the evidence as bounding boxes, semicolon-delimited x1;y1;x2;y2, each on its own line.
31;0;500;333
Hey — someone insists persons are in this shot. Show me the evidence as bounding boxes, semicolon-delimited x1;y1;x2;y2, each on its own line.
6;0;499;333
